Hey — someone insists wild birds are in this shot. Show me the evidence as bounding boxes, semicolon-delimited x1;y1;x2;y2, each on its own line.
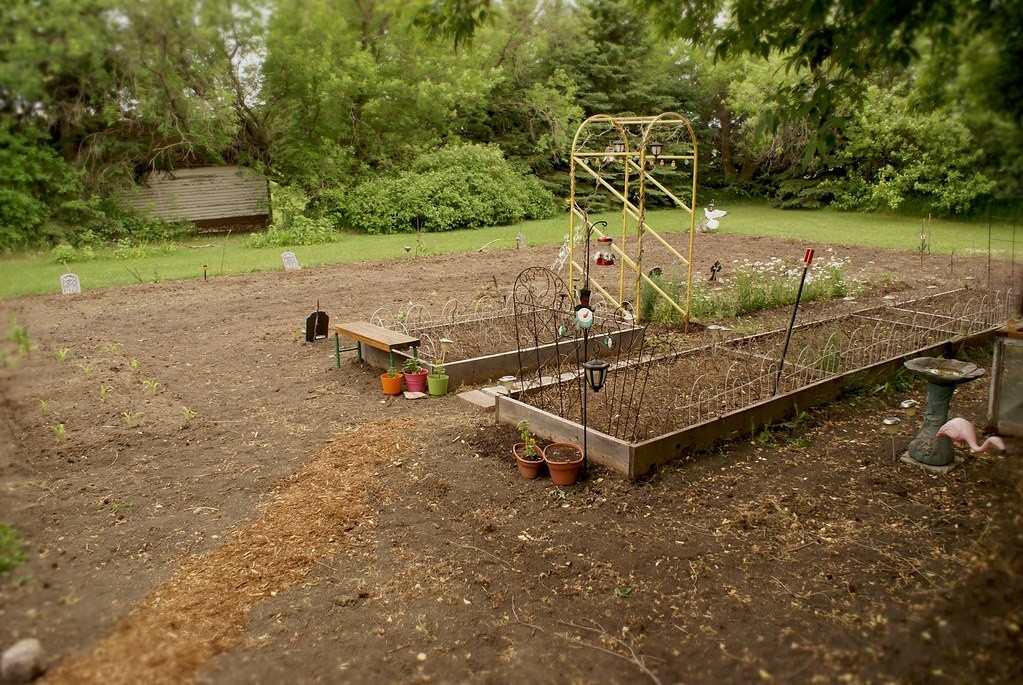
937;417;1007;455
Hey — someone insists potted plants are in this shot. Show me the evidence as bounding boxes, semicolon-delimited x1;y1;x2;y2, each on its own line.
402;358;429;393
381;367;402;394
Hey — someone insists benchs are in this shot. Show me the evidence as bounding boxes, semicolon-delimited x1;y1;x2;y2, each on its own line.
332;321;421;374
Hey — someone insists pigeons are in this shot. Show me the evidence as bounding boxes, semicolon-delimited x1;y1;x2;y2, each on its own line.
704;207;730;230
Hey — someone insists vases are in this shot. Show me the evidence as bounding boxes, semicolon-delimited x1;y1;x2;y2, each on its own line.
513;443;544;478
428;374;449;395
543;442;584;485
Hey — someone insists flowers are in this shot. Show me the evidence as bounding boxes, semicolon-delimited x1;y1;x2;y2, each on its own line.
434;359;446;377
517;419;537;459
